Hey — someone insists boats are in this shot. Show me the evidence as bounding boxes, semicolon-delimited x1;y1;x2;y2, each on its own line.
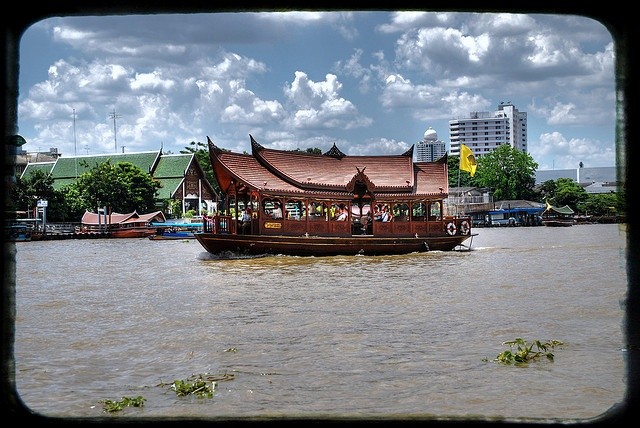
148;222;226;240
542;200;574;227
81;209;167;238
0;225;32;242
194;133;479;258
489;208;545;227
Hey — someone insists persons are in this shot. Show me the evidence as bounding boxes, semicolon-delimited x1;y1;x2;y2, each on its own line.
374;207;381;221
378;206;393;223
337;207;347;221
242;208;251;227
268;203;282;219
363;212;372;229
332;210;340;221
